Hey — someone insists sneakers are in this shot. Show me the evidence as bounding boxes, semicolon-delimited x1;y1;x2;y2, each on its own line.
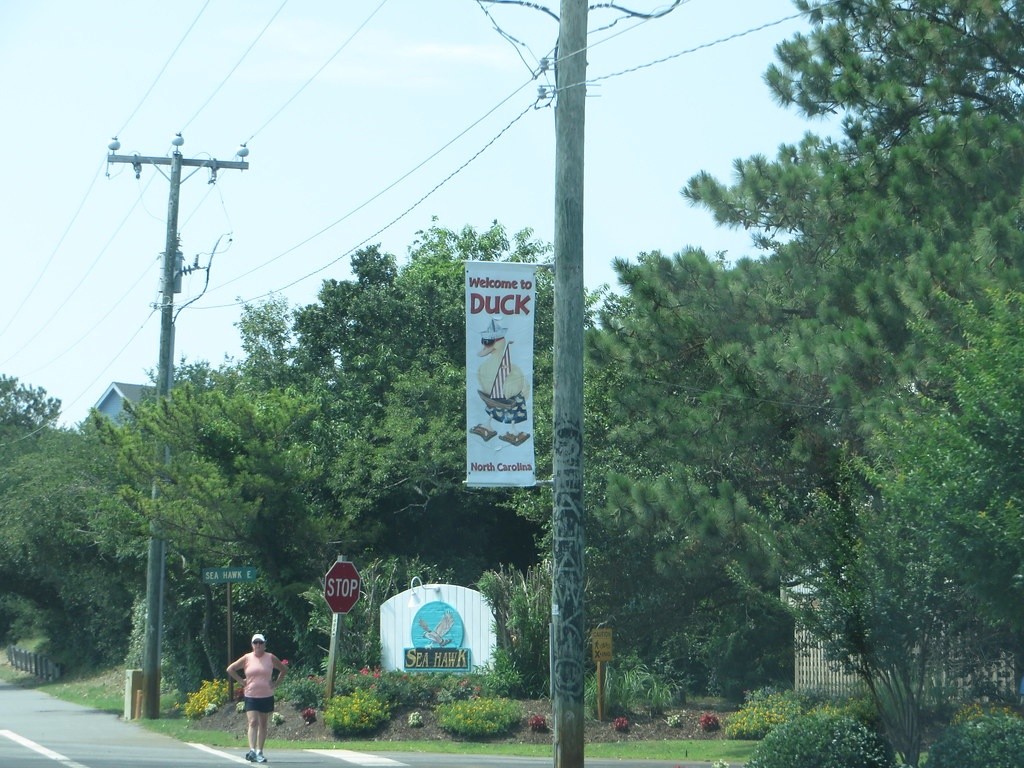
245;749;258;762
256;750;267;763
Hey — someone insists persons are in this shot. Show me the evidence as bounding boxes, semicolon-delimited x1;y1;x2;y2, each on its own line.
227;634;287;762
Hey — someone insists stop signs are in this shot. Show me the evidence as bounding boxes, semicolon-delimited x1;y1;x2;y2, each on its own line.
324;559;362;615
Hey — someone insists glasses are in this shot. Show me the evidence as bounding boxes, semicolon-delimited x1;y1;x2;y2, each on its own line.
481;336;504;345
253;641;265;644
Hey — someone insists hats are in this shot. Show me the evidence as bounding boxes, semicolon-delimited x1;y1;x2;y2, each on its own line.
252;634;266;642
